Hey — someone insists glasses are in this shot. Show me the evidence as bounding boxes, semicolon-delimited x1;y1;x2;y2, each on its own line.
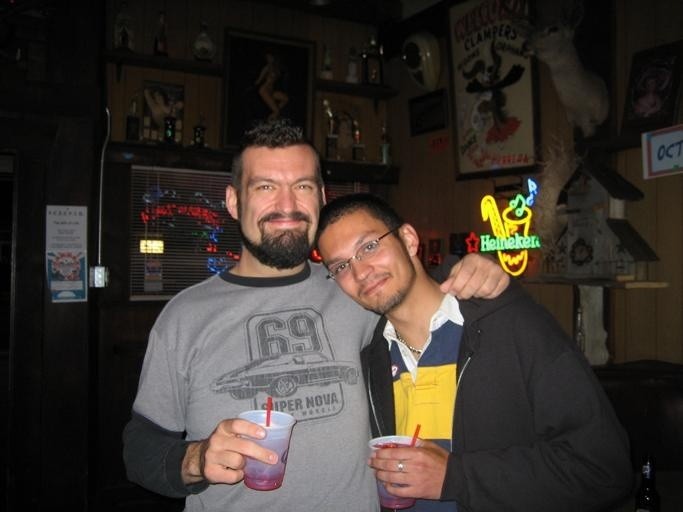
326;226;398;281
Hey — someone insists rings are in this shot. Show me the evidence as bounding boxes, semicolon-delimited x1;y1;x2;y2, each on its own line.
397;460;404;471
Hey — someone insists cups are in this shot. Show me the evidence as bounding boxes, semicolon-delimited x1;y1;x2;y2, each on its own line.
236;409;297;492
368;434;423;510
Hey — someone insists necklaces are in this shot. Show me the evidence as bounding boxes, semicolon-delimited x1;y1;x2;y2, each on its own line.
393;331;425;355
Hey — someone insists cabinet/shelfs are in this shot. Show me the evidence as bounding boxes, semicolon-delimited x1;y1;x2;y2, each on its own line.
101;46;402;186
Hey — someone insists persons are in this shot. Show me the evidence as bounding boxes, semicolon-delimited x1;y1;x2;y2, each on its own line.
118;119;515;512
312;190;632;511
253;48;291;121
140;88;185;128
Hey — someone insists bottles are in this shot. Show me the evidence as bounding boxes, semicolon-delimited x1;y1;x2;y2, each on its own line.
322;31;383;86
114;0;216;62
125;96;205;147
633;453;661;512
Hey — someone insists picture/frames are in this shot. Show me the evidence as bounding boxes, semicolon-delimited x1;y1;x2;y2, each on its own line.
220;26;315;149
619;38;680;148
406;0;544;184
